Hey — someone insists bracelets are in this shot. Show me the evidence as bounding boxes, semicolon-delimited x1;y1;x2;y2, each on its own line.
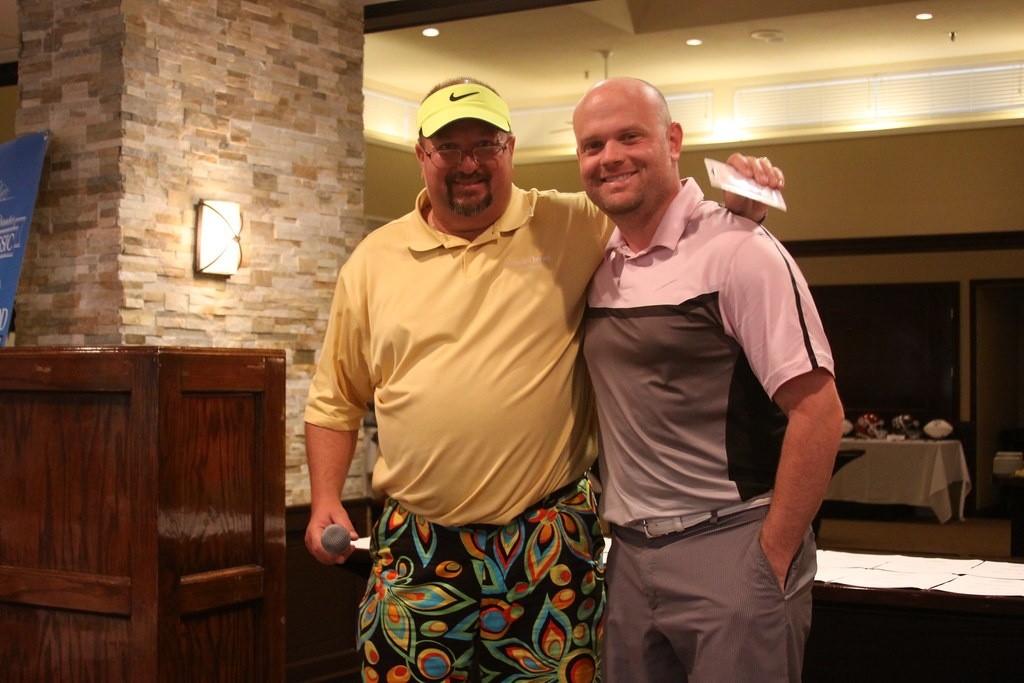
759;210;768;223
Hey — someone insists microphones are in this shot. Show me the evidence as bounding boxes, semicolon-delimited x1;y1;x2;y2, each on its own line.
321;523;350;555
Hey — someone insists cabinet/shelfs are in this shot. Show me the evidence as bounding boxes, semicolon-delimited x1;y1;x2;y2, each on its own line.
1;342;290;682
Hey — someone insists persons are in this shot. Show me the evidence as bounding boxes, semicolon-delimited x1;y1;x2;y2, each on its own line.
304;77;783;683
572;77;845;683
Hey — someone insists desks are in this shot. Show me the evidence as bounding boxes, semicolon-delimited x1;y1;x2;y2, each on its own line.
823;440;973;525
813;547;1024;614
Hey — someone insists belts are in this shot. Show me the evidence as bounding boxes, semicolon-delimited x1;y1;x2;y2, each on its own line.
625;487;776;540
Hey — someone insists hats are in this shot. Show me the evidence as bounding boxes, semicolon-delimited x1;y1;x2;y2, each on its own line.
416;83;512;138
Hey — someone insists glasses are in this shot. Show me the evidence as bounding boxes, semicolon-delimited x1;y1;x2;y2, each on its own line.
420;137;511;168
